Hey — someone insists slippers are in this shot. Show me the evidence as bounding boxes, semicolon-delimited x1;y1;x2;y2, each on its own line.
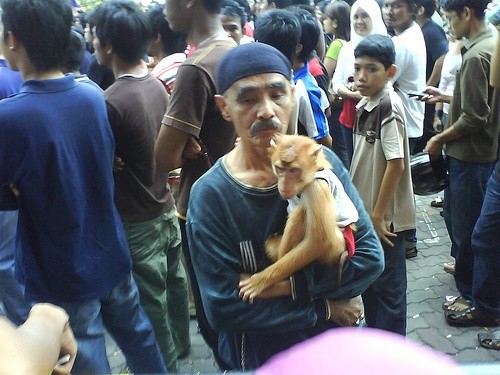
442;295;472;312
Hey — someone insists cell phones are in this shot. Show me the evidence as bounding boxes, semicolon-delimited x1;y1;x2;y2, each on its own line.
407;90;430;97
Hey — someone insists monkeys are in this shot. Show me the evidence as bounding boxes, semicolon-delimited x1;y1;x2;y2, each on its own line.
238;131;349;303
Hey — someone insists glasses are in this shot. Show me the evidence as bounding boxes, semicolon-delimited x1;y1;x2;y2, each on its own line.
446;14;457;22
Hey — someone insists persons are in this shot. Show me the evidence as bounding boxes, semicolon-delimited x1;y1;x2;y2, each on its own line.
1;0;239;375
346;35;417;334
187;43;385;373
419;1;500;348
240;0;430;148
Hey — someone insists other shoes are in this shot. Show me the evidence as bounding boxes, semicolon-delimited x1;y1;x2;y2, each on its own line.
443;262;456;276
430;201;444;206
405;247;418;258
431;179;447;190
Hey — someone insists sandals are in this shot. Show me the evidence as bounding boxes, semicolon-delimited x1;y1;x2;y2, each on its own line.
479;330;500;350
444;305;500;327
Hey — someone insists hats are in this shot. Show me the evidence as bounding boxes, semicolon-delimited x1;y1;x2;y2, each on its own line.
216;42;292;94
